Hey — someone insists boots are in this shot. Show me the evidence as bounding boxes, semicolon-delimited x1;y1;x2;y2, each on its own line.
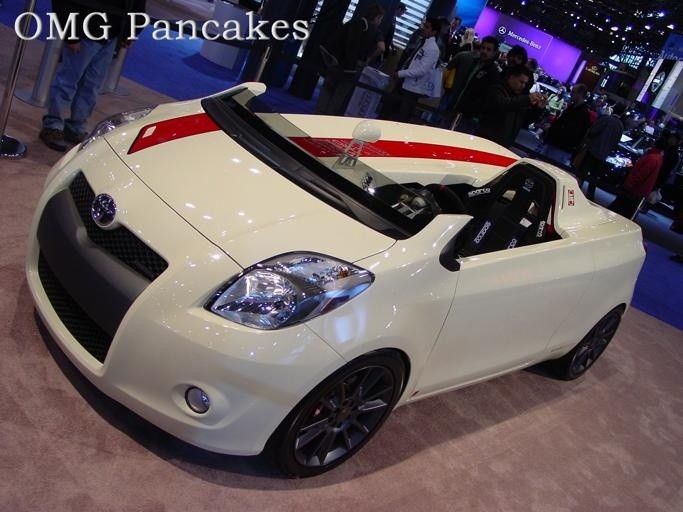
535;128;543;140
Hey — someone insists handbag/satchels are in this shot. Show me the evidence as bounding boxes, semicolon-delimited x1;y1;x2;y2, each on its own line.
444;67;456;88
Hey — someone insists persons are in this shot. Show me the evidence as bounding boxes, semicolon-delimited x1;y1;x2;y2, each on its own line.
40;0;146;152
318;2;683;223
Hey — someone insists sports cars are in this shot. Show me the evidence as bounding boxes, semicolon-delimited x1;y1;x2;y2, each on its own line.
25;82;647;475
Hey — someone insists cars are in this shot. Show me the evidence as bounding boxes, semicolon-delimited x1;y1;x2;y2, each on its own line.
530;82;570;102
602;129;683;194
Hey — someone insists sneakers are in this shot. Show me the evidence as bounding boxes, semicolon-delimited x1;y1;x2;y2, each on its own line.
40;127;89;151
